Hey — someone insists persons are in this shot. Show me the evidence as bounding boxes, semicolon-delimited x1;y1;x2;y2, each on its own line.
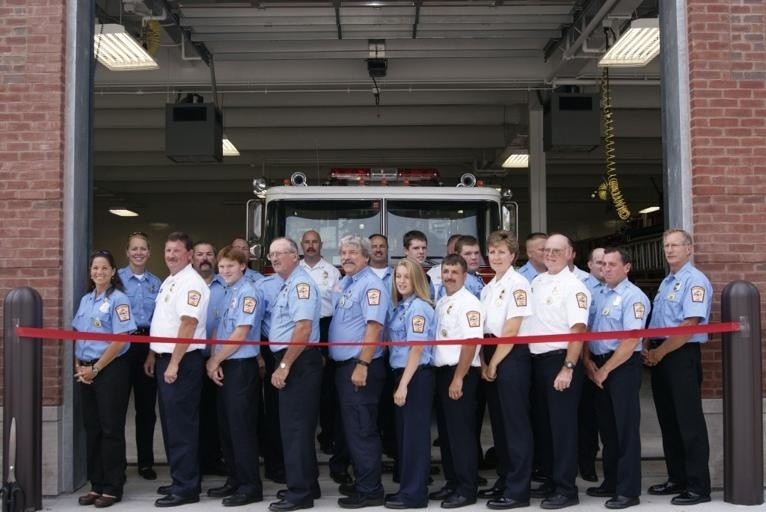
71;229;392;512
578;246;650;509
385;231;591;510
641;231;712;505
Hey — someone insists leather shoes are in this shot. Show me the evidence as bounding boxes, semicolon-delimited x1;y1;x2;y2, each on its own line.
208;482;239;498
265;468;427;511
669;490;713;505
94;495;122;508
78;492;100;506
222;488;263;506
429;468;643;510
138;468;158;480
156;490;200;507
647;479;686;495
158;482;202;495
210;463;227;476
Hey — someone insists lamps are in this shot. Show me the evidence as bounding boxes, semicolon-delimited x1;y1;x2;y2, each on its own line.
91;8;164;74
500;144;530;169
595;4;662;72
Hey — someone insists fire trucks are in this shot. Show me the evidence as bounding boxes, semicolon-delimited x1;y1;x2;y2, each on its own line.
243;165;520;293
